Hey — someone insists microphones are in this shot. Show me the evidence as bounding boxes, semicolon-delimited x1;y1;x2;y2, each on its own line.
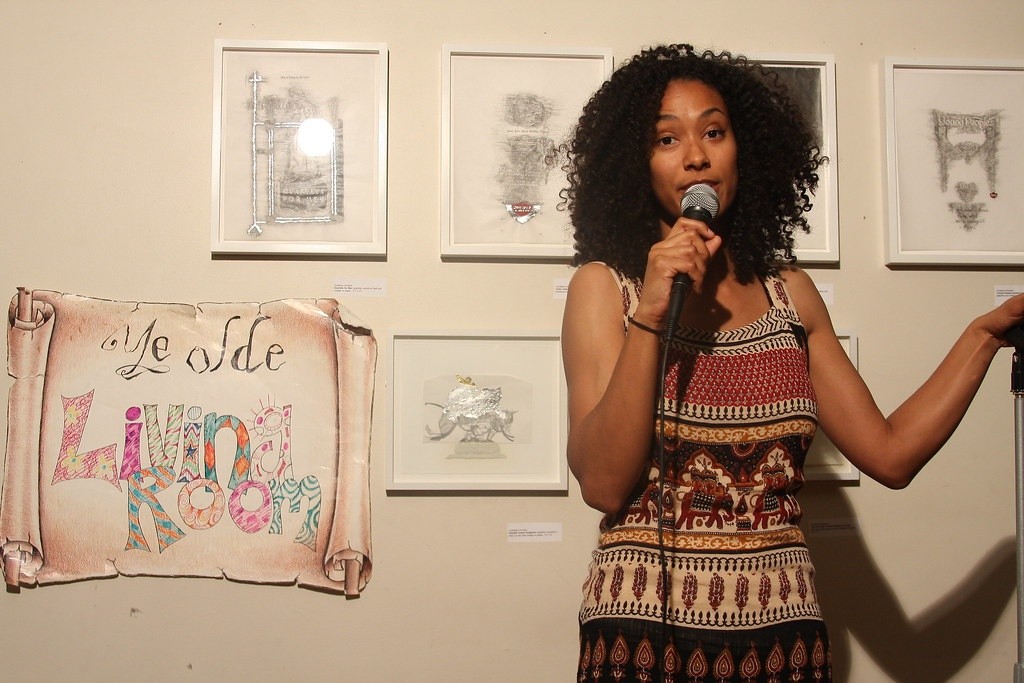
664;183;719;342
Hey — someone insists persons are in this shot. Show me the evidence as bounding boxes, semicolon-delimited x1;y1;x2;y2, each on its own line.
558;42;1024;683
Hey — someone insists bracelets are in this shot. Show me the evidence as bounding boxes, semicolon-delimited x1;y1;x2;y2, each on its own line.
627;315;662;336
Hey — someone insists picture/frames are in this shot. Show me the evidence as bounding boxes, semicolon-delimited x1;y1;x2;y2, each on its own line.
440;44;614;259
713;51;840;263
211;39;388;257
384;328;568;490
884;56;1023;266
803;332;860;481
